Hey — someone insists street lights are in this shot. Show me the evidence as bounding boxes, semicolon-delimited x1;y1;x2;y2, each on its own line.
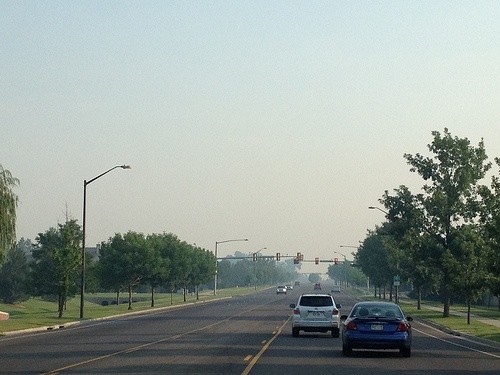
334;251;347;289
214;239;248;295
79;163;133;319
369;206;400;302
339;245;369;291
254;247;267;290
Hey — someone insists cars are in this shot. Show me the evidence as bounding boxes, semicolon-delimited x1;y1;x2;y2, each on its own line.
286;284;294;290
295;281;300;286
339;301;414;357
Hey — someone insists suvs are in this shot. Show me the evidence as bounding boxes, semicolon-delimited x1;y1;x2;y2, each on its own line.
288;293;341;337
277;285;287;294
314;281;321;290
331;285;340;292
